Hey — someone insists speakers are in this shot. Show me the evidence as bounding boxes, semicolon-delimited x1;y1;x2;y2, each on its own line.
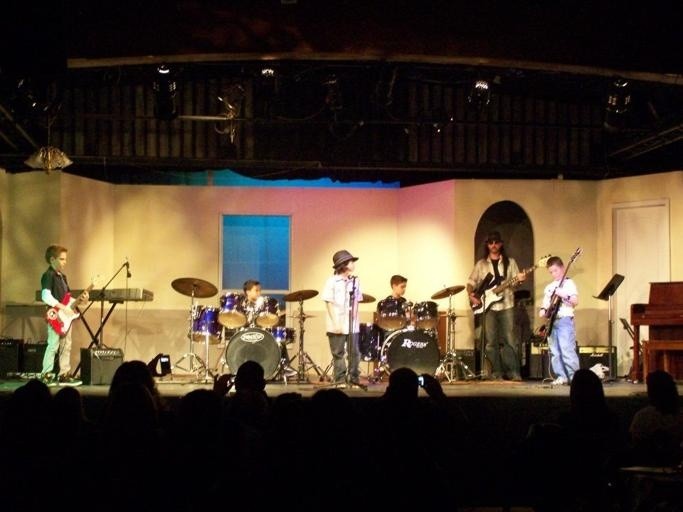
521;338;579;379
0;338;24;372
23;343;59;373
578;345;617;380
80;348;124;385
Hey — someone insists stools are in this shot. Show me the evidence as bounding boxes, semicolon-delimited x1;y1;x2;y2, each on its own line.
641;339;683;387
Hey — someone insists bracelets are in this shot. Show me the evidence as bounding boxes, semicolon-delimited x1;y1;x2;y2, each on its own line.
565;295;570;302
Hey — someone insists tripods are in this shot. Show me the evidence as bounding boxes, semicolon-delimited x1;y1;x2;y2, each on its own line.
434;307;478;385
466;296;497;382
184;331;231;385
323;289;368;391
286;299;332;383
170;286;217;384
318;306;355;383
272;340;299;388
368;330;384;384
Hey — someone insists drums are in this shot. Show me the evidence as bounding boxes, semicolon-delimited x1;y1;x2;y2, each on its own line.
414;302;439;330
270;327;294;345
188;305;221;344
256;298;280;327
225;329;281;379
218;292;247;330
358;322;384;361
380;328;440;377
377;300;406;331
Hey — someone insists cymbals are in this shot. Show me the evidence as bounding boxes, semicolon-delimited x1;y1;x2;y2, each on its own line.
290;314;314;318
431;285;465;299
358;293;376;303
171;277;219;297
283;289;318;301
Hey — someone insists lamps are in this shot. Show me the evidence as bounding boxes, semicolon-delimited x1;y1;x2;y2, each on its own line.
24;111;74;173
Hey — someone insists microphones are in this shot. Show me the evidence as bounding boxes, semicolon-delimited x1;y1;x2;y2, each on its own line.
348;275;358;280
125;259;131;278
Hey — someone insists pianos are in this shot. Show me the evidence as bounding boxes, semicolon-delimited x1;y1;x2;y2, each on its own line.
631;282;683;381
35;288;153;301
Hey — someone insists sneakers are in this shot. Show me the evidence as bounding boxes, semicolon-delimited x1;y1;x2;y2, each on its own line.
333;383;346;388
351;384;359;388
41;372;57;387
486;375;502;381
551;376;568;385
58;371;83;387
512;375;522;381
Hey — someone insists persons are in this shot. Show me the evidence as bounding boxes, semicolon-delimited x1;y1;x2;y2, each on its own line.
41;245;83;387
539;257;580;384
1;361;683;512
377;275;417;325
324;249;365;387
238;280;290;362
467;230;527;381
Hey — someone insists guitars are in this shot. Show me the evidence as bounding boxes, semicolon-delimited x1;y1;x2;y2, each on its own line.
537;246;582;346
469;255;552;315
44;284;94;336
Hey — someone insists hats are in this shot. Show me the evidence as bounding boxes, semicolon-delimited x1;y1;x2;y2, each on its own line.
333;250;359;268
485;232;504;242
